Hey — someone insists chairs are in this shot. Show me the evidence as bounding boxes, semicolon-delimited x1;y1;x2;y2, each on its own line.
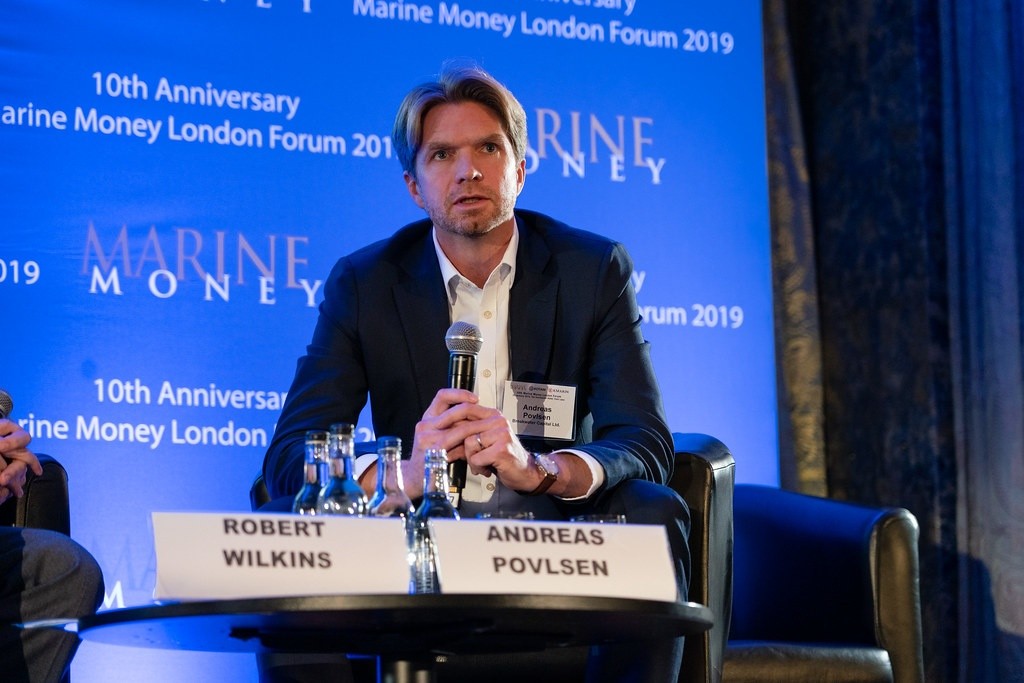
730;482;923;683
671;432;737;683
19;453;72;537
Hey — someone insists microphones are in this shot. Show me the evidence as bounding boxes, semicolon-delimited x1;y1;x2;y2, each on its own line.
0;390;13;419
445;322;483;510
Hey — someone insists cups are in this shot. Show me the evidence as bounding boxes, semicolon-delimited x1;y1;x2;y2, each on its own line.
569;514;625;525
475;511;536;523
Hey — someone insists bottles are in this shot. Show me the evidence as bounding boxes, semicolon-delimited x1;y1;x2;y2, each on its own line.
293;421;461;594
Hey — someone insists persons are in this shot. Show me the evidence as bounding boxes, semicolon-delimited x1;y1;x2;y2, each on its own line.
251;67;694;683
0;415;108;683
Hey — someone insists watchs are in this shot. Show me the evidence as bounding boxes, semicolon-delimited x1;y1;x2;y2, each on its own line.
512;450;561;501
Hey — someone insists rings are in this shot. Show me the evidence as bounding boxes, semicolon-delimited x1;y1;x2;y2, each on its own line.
476;433;485;452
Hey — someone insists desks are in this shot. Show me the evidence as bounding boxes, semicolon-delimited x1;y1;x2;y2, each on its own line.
77;595;714;683
